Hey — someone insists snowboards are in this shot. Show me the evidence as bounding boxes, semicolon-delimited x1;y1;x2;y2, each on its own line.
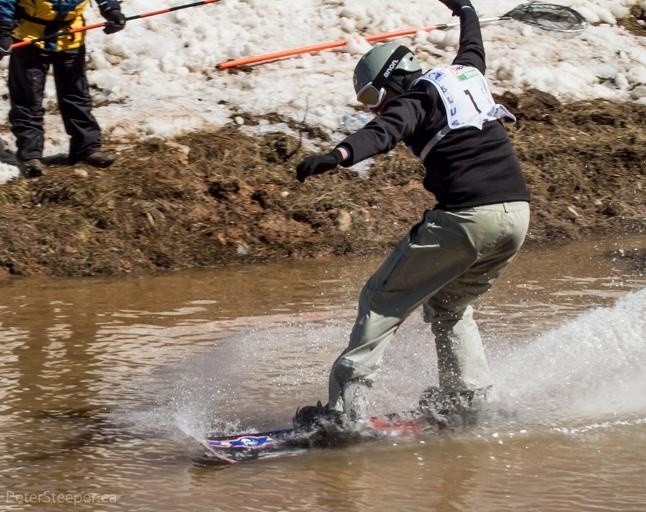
190;407;515;462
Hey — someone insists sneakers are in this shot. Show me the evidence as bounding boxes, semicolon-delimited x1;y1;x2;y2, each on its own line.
83;149;116;169
21;158;45;178
419;385;491;430
296;401;358;432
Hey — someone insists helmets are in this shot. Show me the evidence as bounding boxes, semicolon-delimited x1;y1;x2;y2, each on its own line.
353;43;422;113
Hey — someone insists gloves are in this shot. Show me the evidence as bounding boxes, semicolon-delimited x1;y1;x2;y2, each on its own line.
97;0;126;36
0;26;16;62
438;0;474;17
294;148;344;183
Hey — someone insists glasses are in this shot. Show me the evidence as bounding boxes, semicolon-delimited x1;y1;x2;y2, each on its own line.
355;80;387;112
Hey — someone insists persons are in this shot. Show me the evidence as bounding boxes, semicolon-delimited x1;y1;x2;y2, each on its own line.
0;1;127;179
283;0;534;444
